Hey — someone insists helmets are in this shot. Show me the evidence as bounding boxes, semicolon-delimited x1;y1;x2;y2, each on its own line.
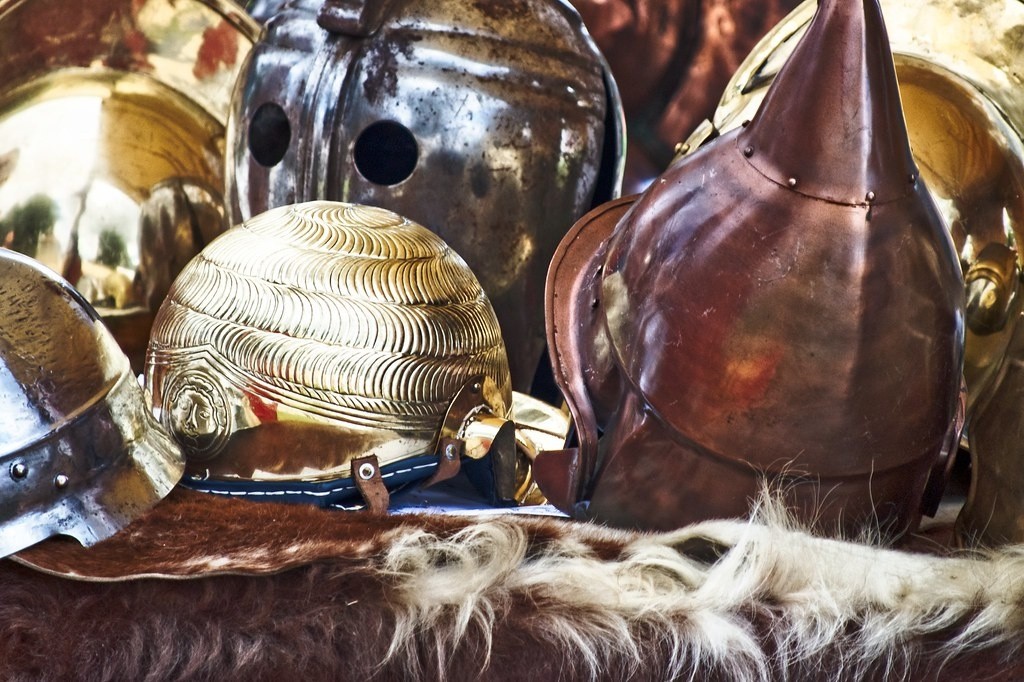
0;66;231;351
0;245;189;564
663;0;1024;495
542;0;973;552
139;199;521;523
226;0;627;408
